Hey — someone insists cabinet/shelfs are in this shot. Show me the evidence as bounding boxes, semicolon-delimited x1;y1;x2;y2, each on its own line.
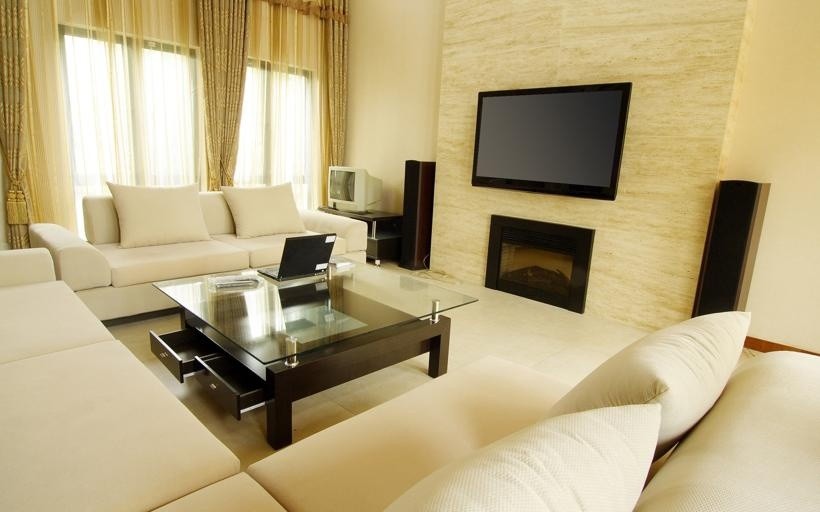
317;203;402;267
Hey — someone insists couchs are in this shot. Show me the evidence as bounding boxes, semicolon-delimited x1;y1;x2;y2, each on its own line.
0;246;819;512
30;192;370;322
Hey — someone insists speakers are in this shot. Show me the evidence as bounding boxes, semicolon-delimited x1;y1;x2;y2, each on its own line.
691;179;771;320
397;159;436;272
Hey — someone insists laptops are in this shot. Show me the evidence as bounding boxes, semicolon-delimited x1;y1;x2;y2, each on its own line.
256;232;337;282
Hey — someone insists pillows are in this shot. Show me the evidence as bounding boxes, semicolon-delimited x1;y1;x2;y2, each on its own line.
105;180;212;247
533;311;752;461
377;403;661;512
220;182;306;238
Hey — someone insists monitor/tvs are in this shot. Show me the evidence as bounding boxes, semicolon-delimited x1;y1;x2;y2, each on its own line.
327;164;382;214
471;81;634;202
